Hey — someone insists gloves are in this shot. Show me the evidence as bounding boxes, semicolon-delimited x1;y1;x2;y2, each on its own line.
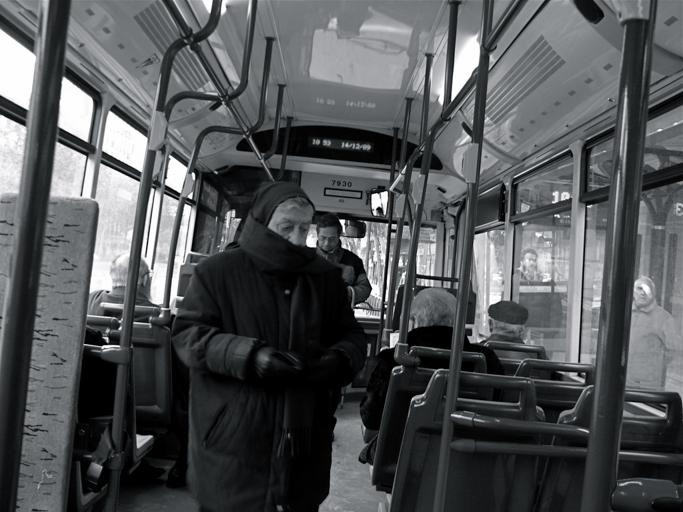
249;345;350;393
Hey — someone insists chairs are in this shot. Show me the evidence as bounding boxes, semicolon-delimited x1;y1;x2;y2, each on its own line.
70;249;208;510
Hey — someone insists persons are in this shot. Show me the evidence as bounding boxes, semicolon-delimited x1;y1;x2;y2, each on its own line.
359;286;507;468
625;275;683;403
85;327;164;480
517;250;549;346
309;212;373;442
170;180;370;512
88;253;177;335
480;300;563;382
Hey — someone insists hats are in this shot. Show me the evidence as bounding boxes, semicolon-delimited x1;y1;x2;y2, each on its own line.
488;300;529;324
